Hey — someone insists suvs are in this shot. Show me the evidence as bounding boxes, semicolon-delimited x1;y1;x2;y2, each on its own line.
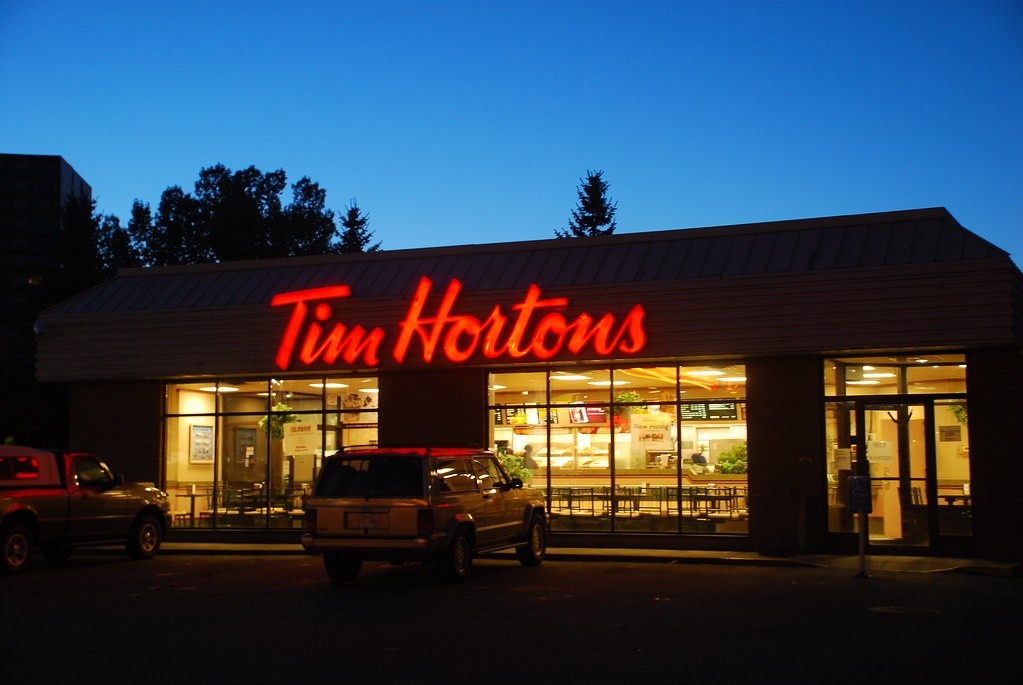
302;444;546;585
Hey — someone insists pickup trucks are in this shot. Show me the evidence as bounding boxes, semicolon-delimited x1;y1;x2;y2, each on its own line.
0;446;172;575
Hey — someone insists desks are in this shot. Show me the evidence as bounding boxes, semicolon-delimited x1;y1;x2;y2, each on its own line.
937;494;971;504
176;494;213;527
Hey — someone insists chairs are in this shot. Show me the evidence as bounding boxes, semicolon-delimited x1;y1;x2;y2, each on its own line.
910;487;924;505
546;485;748;516
174;487;308;528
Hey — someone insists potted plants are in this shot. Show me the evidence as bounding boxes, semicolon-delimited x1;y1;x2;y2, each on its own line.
257;402;301;439
